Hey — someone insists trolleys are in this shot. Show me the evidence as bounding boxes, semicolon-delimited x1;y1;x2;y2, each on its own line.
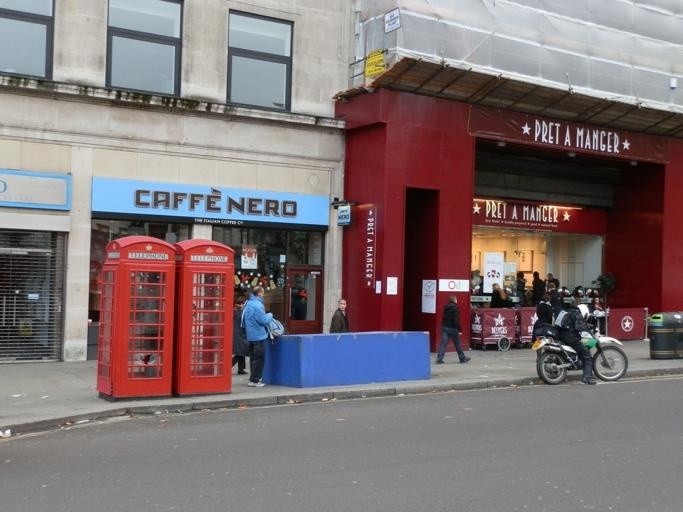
472;307;516;350
517;306;553;348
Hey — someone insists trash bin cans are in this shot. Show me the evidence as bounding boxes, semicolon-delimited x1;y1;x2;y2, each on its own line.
646;311;683;360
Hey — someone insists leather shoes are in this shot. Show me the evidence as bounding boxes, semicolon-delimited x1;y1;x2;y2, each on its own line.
238;368;248;374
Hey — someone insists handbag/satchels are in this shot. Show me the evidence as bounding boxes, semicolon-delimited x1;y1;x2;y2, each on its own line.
234;335;251;357
535;323;561;340
268;318;285;337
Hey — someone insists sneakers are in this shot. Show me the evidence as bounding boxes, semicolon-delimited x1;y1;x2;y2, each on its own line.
461;357;471;363
583;376;597;384
248;379;266;387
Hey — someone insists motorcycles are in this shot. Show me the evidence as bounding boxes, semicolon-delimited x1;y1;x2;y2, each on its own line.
532;309;628;385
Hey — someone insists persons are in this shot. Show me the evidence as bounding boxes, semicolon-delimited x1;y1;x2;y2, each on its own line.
536;293;553;324
470;268;560;308
329;298;348;333
243;286;273;387
434;294;472;364
555;295;598;386
232;294;249;374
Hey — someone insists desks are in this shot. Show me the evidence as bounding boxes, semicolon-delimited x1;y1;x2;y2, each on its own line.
256;330;431;386
